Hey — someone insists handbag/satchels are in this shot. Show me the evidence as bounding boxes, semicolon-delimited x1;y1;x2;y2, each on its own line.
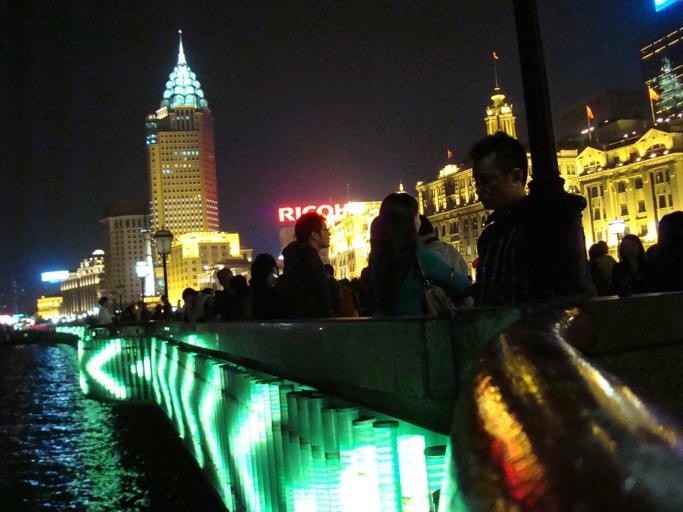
421;281;458;315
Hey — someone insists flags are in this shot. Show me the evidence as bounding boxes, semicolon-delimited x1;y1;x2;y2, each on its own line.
587;107;596;119
649;87;659;100
447;150;454;159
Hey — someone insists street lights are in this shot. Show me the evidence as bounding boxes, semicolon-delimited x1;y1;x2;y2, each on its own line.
151;229;174;312
133;260;147;302
608;214;626;248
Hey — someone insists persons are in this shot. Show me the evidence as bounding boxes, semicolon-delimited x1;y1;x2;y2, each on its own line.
365;192;473;318
463;131;567;308
